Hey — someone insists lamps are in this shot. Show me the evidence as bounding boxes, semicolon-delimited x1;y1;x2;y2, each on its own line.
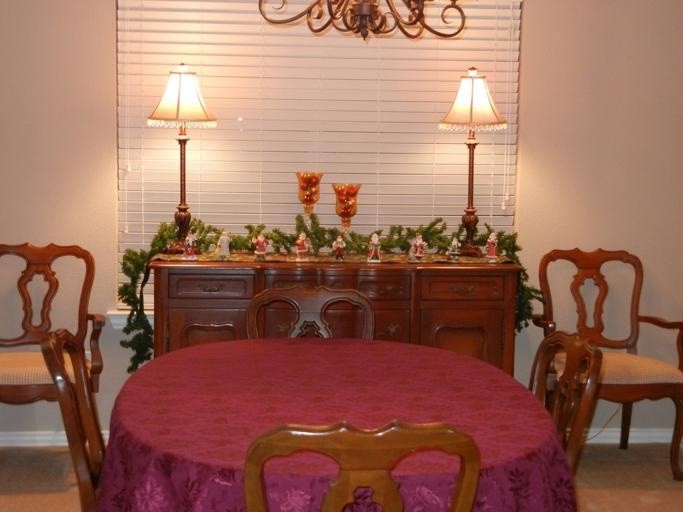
146;66;218;256
0;239;108;512
437;66;509;257
527;334;604;479
532;248;683;481
241;418;481;512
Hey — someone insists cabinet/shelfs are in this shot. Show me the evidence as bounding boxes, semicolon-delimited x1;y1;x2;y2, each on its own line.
147;243;524;378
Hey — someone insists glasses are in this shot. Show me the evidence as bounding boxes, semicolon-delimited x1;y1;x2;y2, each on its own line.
437;66;509;257
146;66;218;256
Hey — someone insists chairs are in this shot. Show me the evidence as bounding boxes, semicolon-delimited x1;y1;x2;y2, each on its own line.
241;418;481;512
532;248;683;481
527;334;604;479
0;239;108;512
